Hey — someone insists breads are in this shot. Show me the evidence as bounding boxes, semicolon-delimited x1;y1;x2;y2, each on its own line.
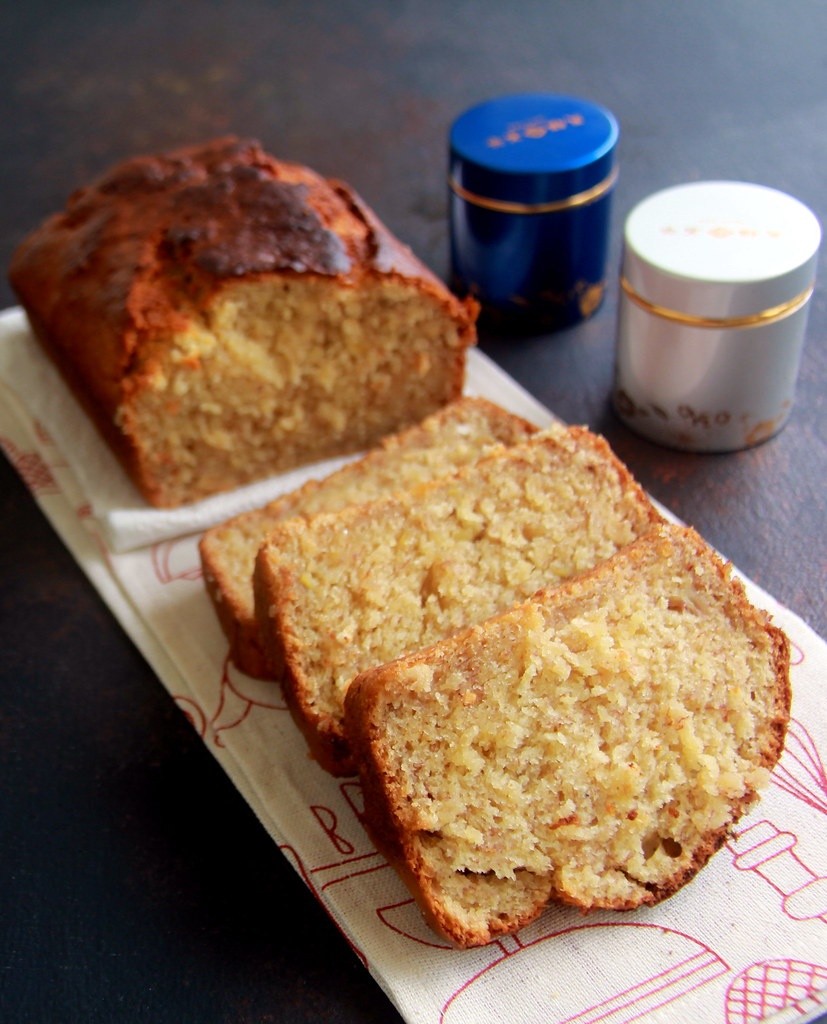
10;138;479;510
199;399;791;949
